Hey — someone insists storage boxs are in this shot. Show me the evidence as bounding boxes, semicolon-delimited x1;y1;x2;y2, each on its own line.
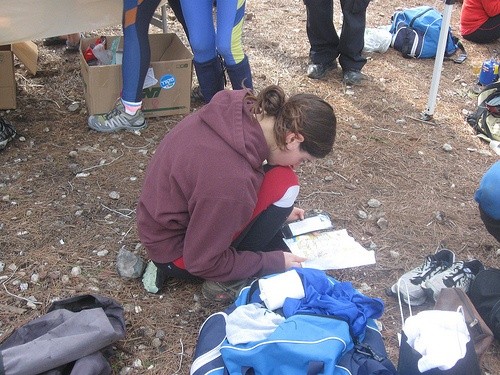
0;41;39;110
79;32;194;117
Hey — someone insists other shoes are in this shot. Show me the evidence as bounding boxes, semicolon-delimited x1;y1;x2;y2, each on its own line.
343;68;362;83
201;278;236;304
307;58;338;78
143;261;166;293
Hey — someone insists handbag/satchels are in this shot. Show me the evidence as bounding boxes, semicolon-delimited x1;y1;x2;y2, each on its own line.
397;278;479;375
467;269;500;343
389;6;466;63
435;287;493;354
189;277;397;375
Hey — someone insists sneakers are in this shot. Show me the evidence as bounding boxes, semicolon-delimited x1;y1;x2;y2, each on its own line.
87;99;148;132
0;118;17;147
422;259;484;304
392;250;455;306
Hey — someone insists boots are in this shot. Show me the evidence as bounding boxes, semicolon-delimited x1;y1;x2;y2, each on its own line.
194;49;225;101
224;55;253;94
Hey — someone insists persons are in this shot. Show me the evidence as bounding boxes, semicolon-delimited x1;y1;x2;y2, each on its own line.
460;0;500;44
136;85;337;303
89;0;254;132
303;0;372;85
